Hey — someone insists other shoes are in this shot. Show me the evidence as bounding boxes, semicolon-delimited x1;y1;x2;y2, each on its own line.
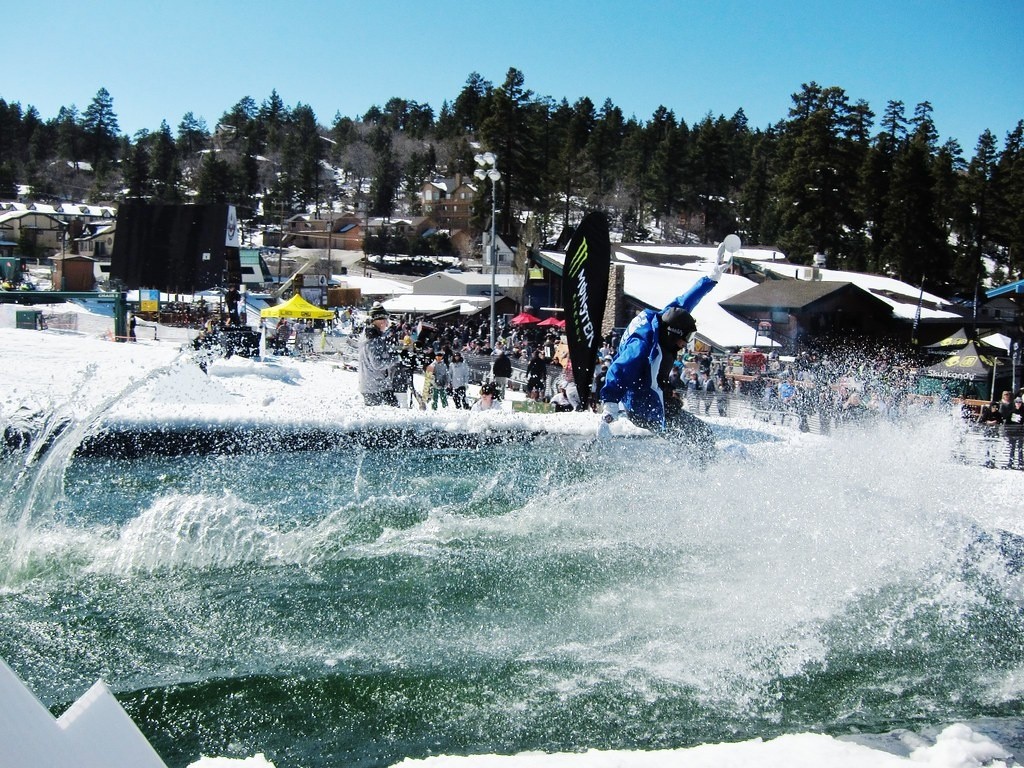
983;462;995;469
1008;462;1024;468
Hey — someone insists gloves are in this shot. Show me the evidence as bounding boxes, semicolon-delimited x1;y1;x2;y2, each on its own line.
400;348;411;360
596;402;619;442
706;242;728;283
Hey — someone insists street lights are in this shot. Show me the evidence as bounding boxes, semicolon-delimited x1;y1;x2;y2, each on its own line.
473;152;501;348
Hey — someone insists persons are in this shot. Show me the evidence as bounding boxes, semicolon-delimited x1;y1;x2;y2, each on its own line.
359;302;409;409
595;243;734;469
271;316;315;356
447;352;471;410
224;284;243;328
587;311;1024;460
431;351;448;410
422;361;442;403
471;382;503;411
319;303;576;398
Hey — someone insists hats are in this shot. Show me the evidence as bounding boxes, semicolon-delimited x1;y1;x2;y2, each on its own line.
370;301;389;324
1014;396;1022;403
989;401;1000;410
435;352;444;356
700;369;710;376
661;306;697;338
454;351;461;357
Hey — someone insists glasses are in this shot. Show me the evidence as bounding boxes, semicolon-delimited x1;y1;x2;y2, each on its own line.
481;389;491;395
684;331;696;343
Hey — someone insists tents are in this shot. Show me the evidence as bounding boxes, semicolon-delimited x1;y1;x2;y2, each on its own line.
919;326;1008;357
912;338;1024;400
260;293;334;348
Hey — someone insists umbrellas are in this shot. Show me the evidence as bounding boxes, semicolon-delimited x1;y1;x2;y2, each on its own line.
556;318;567;327
537;317;560;326
514;313;541;324
511;312;527;321
